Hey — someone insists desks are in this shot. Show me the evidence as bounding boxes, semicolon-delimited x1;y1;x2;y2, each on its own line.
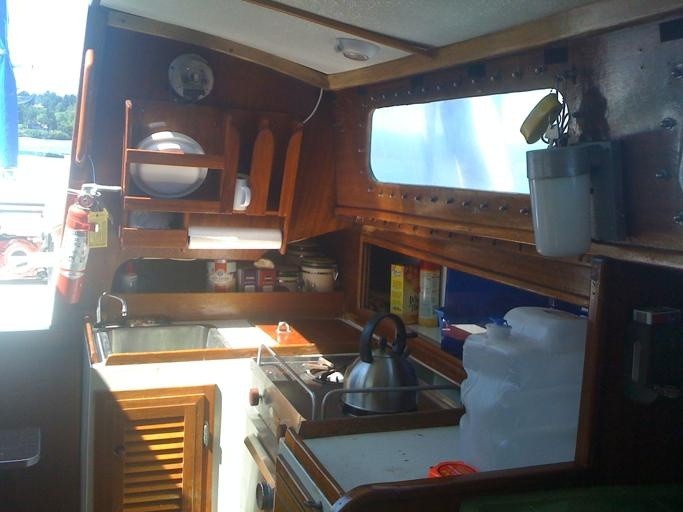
0;425;41;469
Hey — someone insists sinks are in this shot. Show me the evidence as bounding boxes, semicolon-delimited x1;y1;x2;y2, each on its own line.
96;321;233;363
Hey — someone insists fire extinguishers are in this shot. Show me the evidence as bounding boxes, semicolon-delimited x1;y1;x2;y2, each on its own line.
56;183;122;304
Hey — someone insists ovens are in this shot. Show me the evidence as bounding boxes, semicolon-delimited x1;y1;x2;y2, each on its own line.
234;416;323;511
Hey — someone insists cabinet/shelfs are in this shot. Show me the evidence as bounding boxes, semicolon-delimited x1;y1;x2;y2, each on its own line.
271;206;683;512
118;98;303;261
93;383;219;512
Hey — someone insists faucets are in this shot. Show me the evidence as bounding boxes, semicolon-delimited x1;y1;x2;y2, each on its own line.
95;290;128;325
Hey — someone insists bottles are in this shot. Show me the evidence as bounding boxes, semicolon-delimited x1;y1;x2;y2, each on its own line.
120;262;138;294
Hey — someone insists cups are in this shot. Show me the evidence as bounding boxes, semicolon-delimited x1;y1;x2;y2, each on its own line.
232;173;252;210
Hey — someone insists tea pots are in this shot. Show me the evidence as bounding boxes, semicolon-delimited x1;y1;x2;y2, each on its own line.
340;313;420;416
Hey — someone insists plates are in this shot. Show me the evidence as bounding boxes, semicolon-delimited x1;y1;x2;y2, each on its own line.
168;53;214;103
129;131;209;199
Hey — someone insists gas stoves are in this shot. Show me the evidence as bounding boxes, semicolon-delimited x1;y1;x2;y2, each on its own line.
246;356;464;442
